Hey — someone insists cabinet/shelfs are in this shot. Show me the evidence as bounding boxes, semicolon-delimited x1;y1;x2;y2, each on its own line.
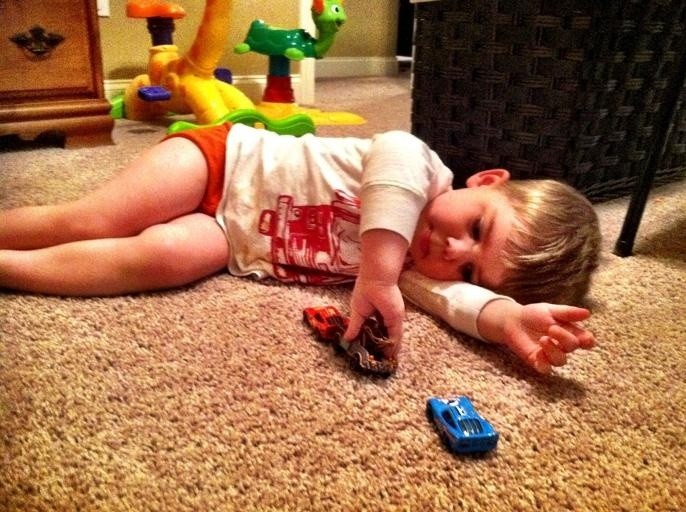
0;1;120;150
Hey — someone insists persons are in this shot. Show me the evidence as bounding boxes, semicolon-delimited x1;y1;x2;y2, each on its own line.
0;117;605;377
